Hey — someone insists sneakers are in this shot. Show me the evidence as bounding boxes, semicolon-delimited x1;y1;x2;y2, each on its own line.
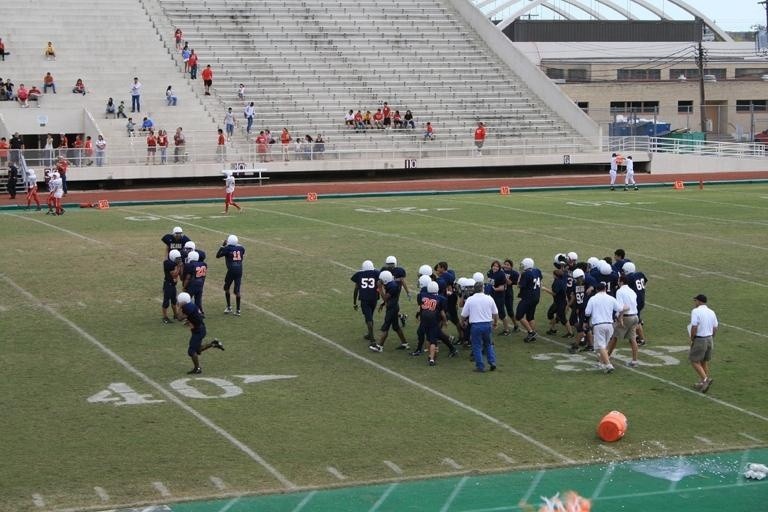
604;363;615;374
448;336;496;371
636;336;645;345
694;378;713;391
396;343;439;365
627;361;638;367
187;368;201;374
212;338;225;351
546;328;594;354
365;335;383;352
502;326;537;342
224;307;240;316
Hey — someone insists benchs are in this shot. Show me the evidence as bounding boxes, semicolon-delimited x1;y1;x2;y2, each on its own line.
0;1;605;168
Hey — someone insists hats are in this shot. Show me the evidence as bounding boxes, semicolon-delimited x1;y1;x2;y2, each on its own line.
693;295;706;303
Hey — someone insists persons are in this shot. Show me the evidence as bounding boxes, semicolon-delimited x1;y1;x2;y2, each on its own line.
220;170;242;215
0;27;326;166
6;155;70;216
703;116;713;133
216;234;245;317
475;122;486;153
176;292;225;373
345;102;414;129
620;156;639;191
423;122;434;141
687;294;719;393
160;227;208;326
348;248;648;375
608;152;619;190
232;165;246;186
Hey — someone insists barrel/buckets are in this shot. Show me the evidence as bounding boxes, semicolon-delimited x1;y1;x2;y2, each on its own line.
598;410;629;442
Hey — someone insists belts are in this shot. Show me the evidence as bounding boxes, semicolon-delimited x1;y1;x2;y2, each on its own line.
623;314;637;316
696;336;711;338
593;323;612;326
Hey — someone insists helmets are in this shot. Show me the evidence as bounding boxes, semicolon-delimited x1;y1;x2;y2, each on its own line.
554;252;635;279
379;270;393;285
521;257;534;272
418;265;439;293
455;272;484;295
169;227;199;264
227;235;238;246
49;173;60;179
362;260;374;272
224;170;233;177
178;292;191;303
386;256;397;268
